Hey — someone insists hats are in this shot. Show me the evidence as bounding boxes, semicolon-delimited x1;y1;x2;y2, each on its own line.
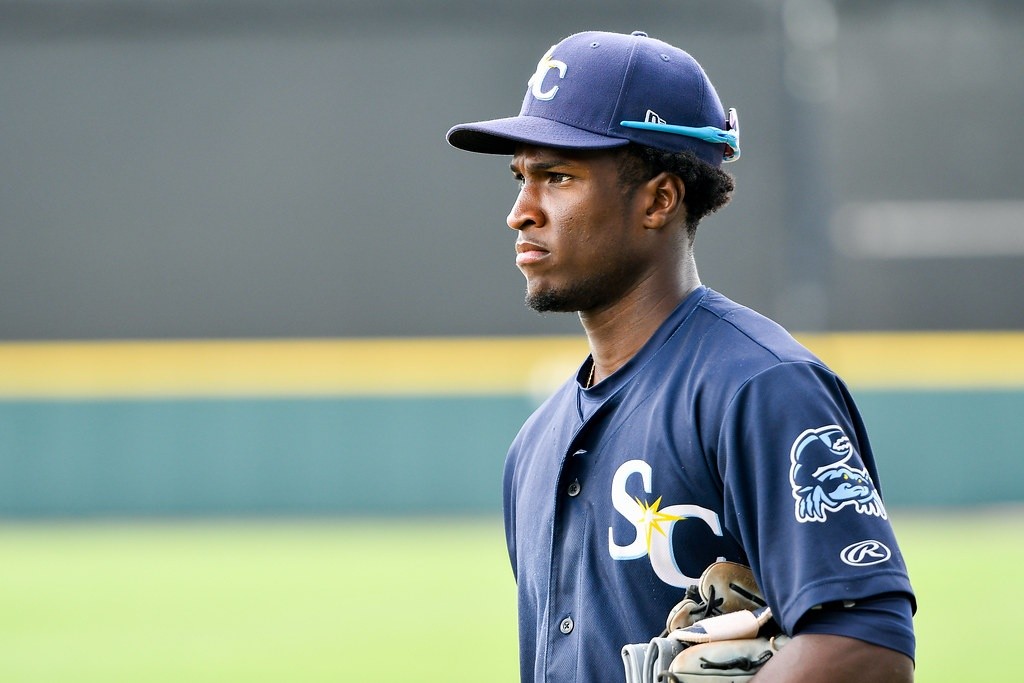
446;31;729;169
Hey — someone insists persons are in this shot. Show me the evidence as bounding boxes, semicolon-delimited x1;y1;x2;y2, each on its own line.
448;32;917;683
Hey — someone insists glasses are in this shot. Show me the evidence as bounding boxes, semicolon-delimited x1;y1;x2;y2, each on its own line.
620;108;741;163
